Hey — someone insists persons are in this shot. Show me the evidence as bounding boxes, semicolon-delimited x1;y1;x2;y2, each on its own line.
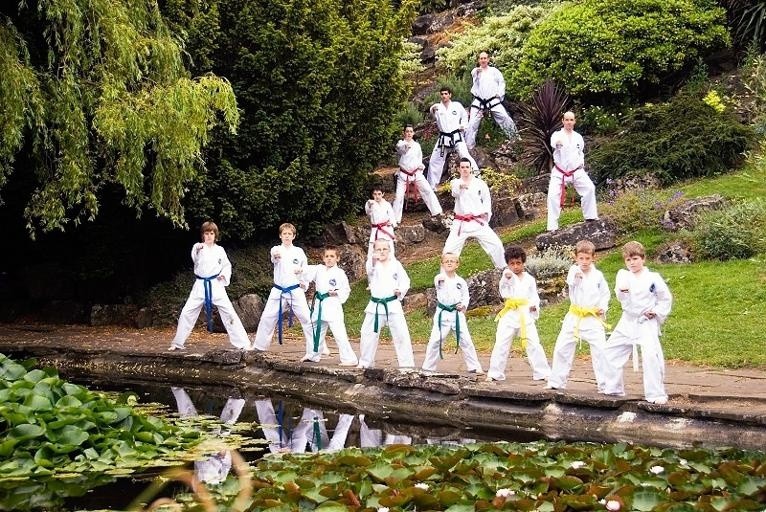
545;111;600;235
424;86;479;186
421;252;483;374
300;246;357;366
442;158;508;267
173;385;478;485
393;124;445;223
547;241;610;393
251;223;313;351
485;247;551;383
366;187;397;287
463;49;524;150
602;240;671;402
356;237;414;367
168;222;251;349
